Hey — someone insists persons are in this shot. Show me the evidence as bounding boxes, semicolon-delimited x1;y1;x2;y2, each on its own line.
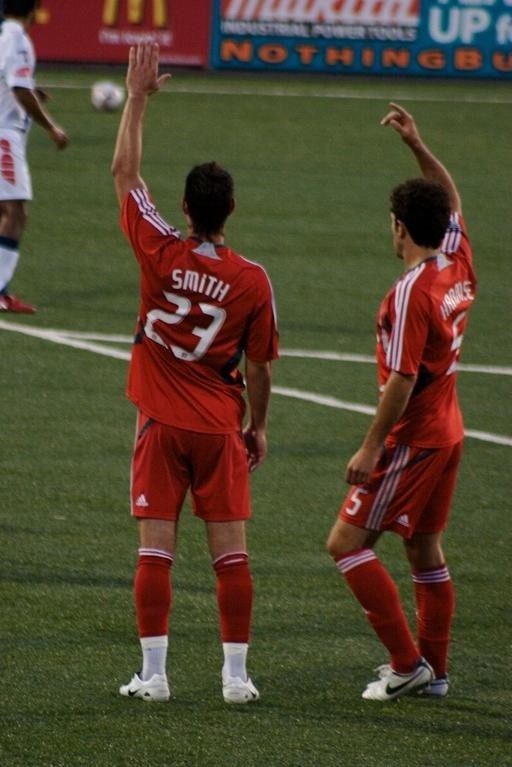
110;37;280;706
0;0;70;313
326;102;479;701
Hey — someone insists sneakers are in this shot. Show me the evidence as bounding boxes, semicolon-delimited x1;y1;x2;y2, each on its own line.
0;292;38;313
222;674;259;704
361;656;449;701
118;671;170;702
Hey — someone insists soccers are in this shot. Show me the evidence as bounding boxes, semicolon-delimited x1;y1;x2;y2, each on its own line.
91;82;122;112
